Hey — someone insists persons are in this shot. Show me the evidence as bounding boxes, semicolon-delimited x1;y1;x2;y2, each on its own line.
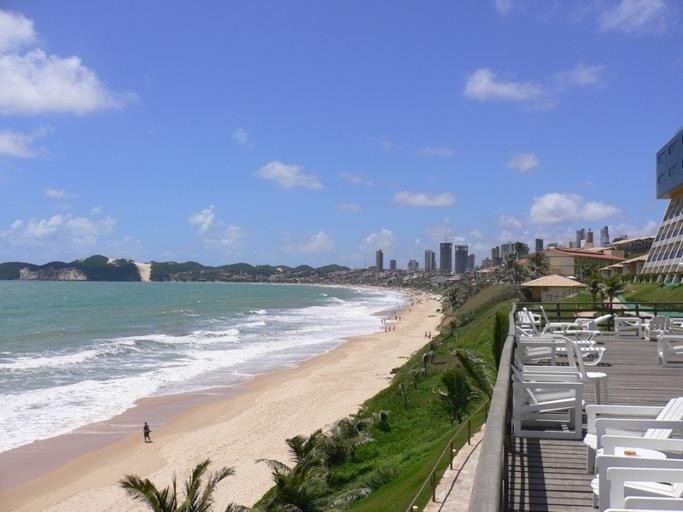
143;422;151;442
380;288;434;339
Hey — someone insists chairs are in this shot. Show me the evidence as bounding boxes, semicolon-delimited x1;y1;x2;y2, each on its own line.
583;394;683;512
614;314;683;368
512;302;614;440
